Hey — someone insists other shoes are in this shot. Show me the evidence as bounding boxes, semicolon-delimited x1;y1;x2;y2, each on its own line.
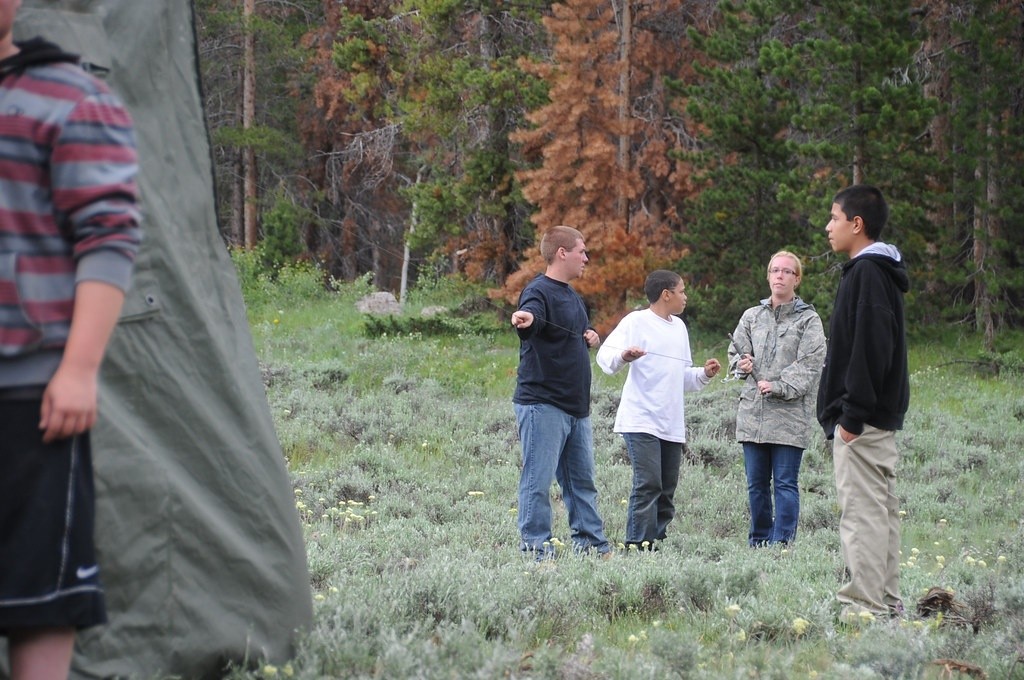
624;541;662;557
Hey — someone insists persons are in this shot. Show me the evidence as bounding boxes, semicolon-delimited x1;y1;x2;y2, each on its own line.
0;0;140;680
597;269;720;551
815;185;910;624
728;251;827;547
512;227;610;561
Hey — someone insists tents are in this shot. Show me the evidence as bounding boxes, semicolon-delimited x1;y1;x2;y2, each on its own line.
3;0;310;680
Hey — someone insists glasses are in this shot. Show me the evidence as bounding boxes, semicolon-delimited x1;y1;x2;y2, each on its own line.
769;267;800;277
